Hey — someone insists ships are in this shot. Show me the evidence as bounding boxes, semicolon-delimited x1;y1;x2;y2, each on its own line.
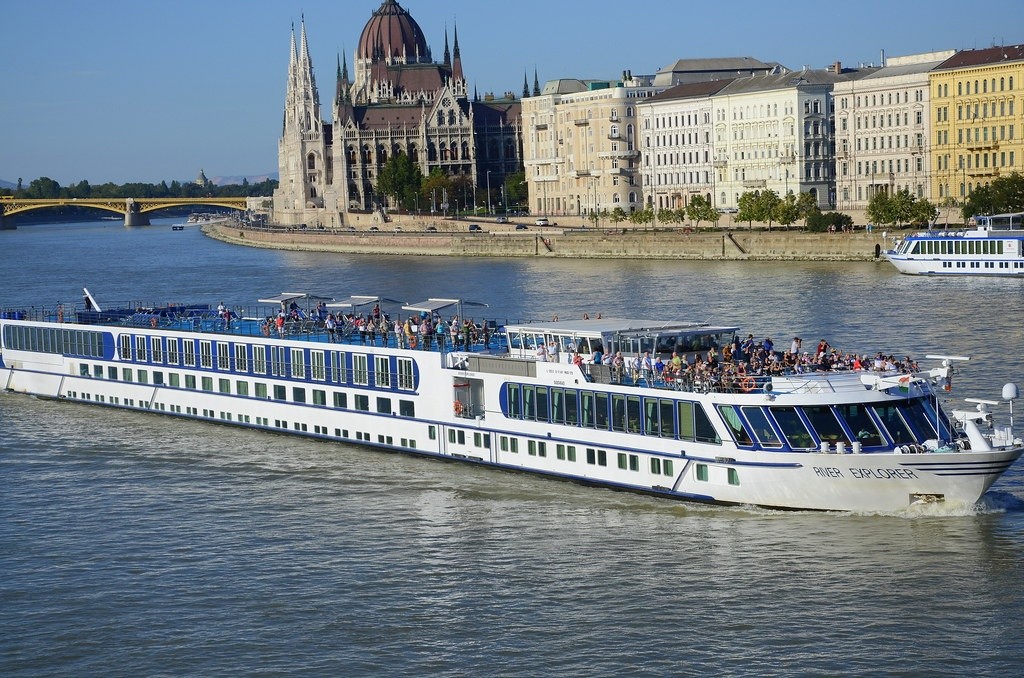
0;285;1023;518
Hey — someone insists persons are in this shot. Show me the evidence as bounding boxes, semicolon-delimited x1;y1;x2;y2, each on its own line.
262;300;491;351
534;311;922;393
223;308;232;330
217;300;225;320
827;223;873;234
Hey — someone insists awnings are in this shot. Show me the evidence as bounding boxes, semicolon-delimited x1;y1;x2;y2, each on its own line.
326;294;404;321
402;298;489;322
257;291;333;312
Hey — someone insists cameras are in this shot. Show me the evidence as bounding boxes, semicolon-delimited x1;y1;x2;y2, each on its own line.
825;342;831;348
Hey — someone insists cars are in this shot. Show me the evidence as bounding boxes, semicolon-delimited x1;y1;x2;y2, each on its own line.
427;227;437;230
318;227;324;229
370;226;379;230
496;217;507;223
348;227;356;231
516;224;528;229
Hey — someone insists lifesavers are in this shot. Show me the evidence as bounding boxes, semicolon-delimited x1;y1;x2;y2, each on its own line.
262;325;270;336
454;400;462;414
408;336;417;348
151;317;157;328
742;377;756;392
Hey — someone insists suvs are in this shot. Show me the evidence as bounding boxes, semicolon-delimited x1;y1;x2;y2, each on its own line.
534;217;549;226
300;223;307;229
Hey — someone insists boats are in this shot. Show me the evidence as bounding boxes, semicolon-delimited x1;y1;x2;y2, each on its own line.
172;223;184;230
187;210;227;223
881;212;1024;278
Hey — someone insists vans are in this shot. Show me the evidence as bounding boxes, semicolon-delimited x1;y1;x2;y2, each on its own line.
394;225;403;231
469;225;482;230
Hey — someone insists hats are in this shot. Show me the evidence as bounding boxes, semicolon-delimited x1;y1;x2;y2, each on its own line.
551;341;554;343
794;337;800;340
819;352;826;358
804;352;809;355
539;342;544;344
758;340;762;343
764;336;770;339
830;347;838;353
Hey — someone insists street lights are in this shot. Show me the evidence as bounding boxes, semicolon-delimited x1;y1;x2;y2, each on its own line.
412;199;417;216
516;203;520;220
482;201;487;224
430;191;434;218
394;191;399;213
353;192;357;200
415;191;418;211
382;190;386;215
368;192;373;211
454;199;459;222
486;171;492;215
